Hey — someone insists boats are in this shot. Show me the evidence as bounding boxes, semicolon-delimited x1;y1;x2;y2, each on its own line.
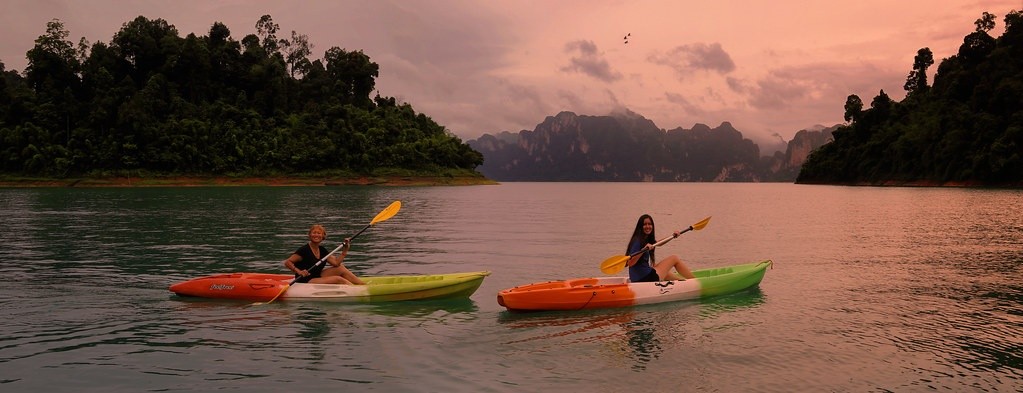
501;258;774;315
168;257;493;303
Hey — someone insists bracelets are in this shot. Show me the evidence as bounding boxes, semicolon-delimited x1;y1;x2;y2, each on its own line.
293;267;296;272
342;253;346;256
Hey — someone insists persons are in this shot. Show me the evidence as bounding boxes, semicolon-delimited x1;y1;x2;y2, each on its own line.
285;224;367;285
625;214;696;283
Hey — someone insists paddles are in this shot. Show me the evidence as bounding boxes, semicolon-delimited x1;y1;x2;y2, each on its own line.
268;199;402;305
600;215;713;275
